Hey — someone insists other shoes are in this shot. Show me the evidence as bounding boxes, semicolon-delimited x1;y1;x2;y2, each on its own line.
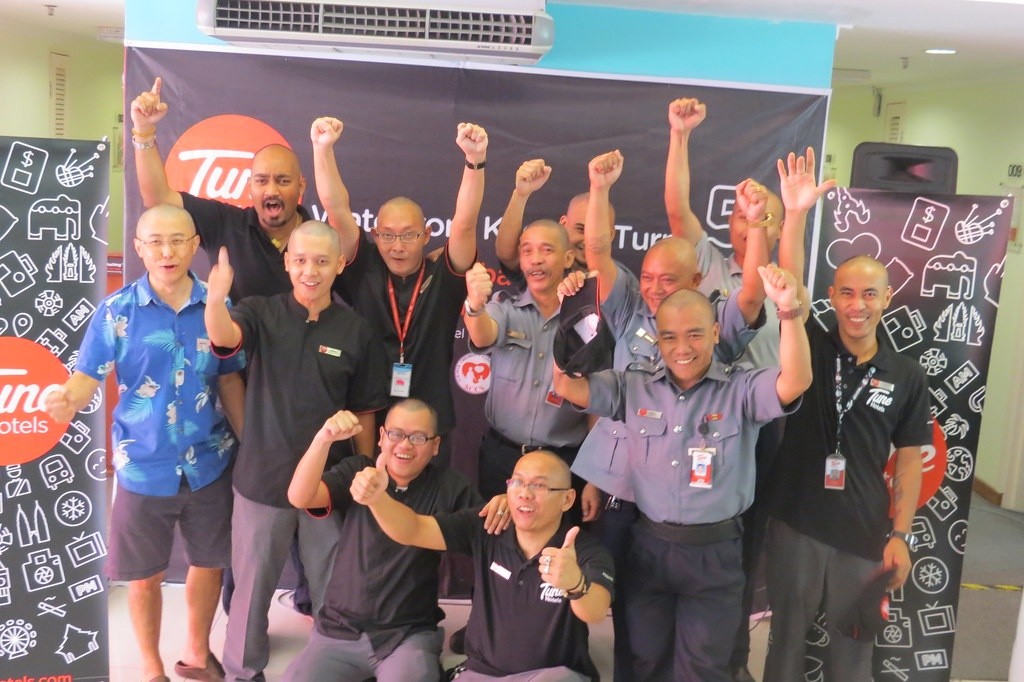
449;623;467;655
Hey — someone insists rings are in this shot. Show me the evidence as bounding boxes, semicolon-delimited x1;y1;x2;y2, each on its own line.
544;566;550;574
544;556;551;566
496;508;504;517
781;272;785;277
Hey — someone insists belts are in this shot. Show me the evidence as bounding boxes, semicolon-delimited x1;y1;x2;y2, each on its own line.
637;514;744;545
488;428;577;460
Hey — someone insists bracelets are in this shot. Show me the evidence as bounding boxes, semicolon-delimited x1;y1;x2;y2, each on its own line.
746;212;775;228
132;135;158;149
465;298;487;317
562;574;591;600
776;301;803;320
132;126;156;138
465;157;487;170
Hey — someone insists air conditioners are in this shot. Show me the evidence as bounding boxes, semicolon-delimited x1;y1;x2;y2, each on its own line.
197;1;554;68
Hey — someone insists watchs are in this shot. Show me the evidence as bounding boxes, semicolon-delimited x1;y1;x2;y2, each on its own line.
885;529;914;552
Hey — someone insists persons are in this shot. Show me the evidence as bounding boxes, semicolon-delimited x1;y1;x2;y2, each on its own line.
202;220;378;682
282;399;511;682
131;77;814;616
558;262;814;682
695;464;706;475
777;147;932;682
45;204;247;682
350;452;614;682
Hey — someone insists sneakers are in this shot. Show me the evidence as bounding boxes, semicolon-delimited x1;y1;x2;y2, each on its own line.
172;654;226;682
148;675;171;682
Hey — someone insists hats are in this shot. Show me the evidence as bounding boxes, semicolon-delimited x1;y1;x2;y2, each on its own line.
549;277;616;380
835;568;896;645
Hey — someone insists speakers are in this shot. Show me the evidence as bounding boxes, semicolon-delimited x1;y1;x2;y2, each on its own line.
849;141;958;196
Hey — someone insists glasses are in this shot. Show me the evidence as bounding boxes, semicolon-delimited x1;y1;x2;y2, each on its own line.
375;228;424;244
504;478;569;496
384;426;437;447
134;235;196;249
